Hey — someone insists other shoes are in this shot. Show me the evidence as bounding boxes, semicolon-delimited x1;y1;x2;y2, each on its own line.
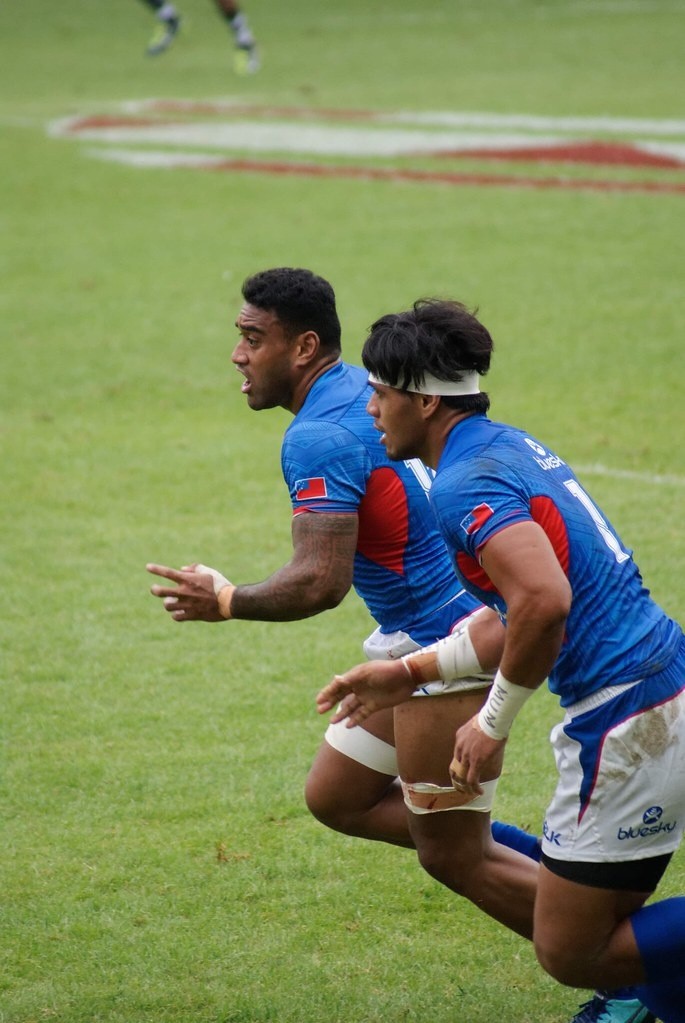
146;16;180;51
235;25;254;52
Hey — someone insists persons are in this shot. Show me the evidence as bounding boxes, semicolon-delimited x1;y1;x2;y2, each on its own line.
141;0;258;75
146;268;542;941
317;300;685;1023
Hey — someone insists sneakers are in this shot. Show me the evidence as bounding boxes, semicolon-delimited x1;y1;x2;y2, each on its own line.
571;991;656;1023
